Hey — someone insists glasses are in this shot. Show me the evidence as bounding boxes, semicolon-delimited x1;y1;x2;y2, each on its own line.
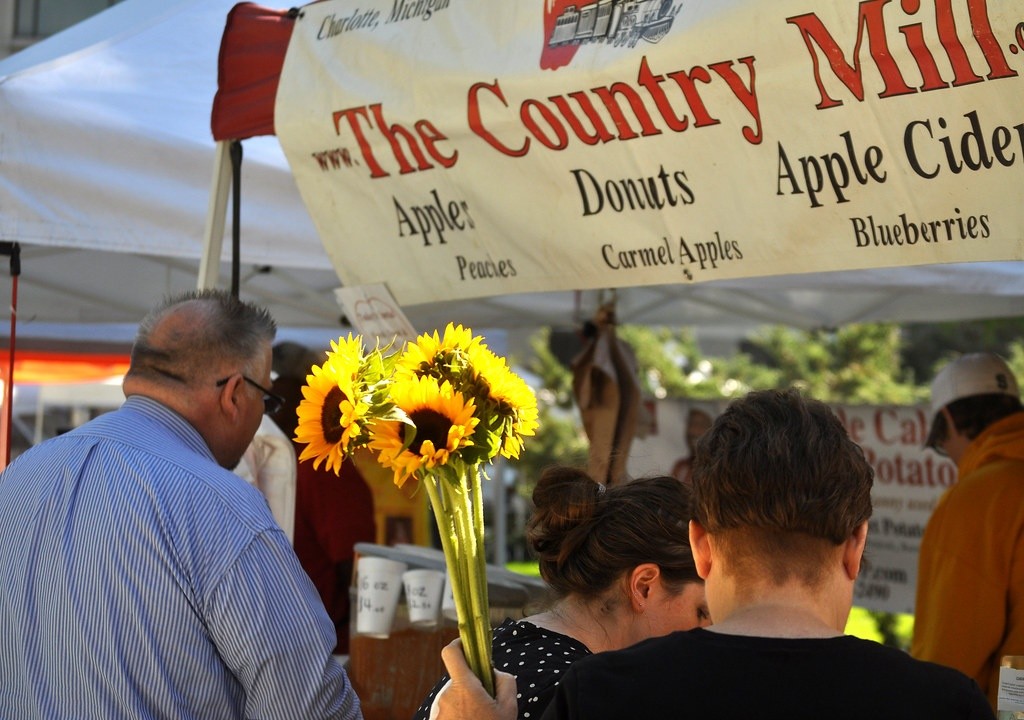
215;374;285;413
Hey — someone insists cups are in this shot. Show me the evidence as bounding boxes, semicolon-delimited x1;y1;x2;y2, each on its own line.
356;556;408;639
442;569;458;622
402;569;445;627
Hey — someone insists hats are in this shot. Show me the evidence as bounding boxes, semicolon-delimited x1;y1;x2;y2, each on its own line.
918;353;1019;451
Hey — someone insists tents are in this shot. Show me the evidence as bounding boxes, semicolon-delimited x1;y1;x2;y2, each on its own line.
0;0;1024;331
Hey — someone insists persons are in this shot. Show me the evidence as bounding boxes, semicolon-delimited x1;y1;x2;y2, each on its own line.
907;352;1024;713
671;403;716;483
0;290;364;720
232;341;377;654
413;466;710;720
429;388;996;720
